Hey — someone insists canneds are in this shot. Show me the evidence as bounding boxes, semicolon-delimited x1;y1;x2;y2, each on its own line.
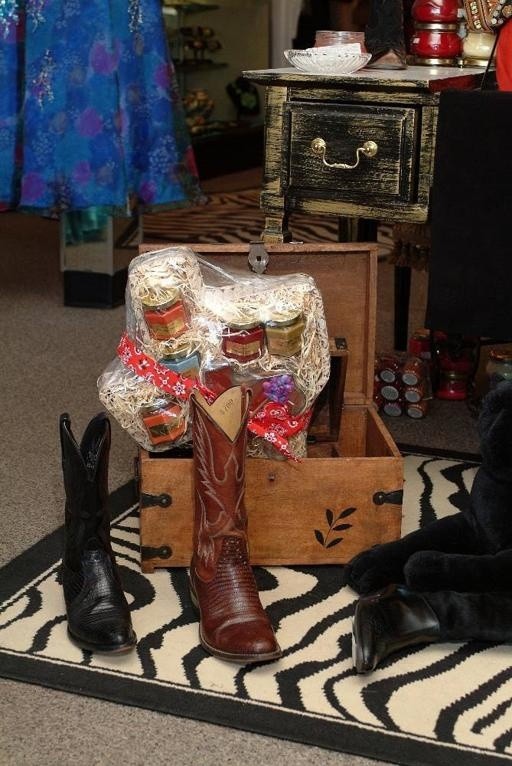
409;0;497;67
484;347;512;389
139;287;306;447
372;328;430;419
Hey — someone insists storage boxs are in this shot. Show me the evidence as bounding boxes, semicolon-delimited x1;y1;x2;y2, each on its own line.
119;234;409;574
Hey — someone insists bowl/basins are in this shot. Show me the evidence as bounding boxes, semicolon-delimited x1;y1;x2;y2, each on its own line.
281;49;374;77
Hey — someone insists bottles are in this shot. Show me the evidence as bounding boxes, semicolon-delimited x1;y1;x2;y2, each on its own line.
313;29;368;69
410;324;512;404
410;0;461;67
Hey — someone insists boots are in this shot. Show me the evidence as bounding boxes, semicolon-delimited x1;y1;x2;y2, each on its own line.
178;384;288;665
50;410;141;656
350;576;448;677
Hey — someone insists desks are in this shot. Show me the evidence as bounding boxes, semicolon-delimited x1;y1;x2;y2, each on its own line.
237;53;497;242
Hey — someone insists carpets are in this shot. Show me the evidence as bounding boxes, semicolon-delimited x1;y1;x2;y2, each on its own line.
115;182;341;248
1;427;512;766
